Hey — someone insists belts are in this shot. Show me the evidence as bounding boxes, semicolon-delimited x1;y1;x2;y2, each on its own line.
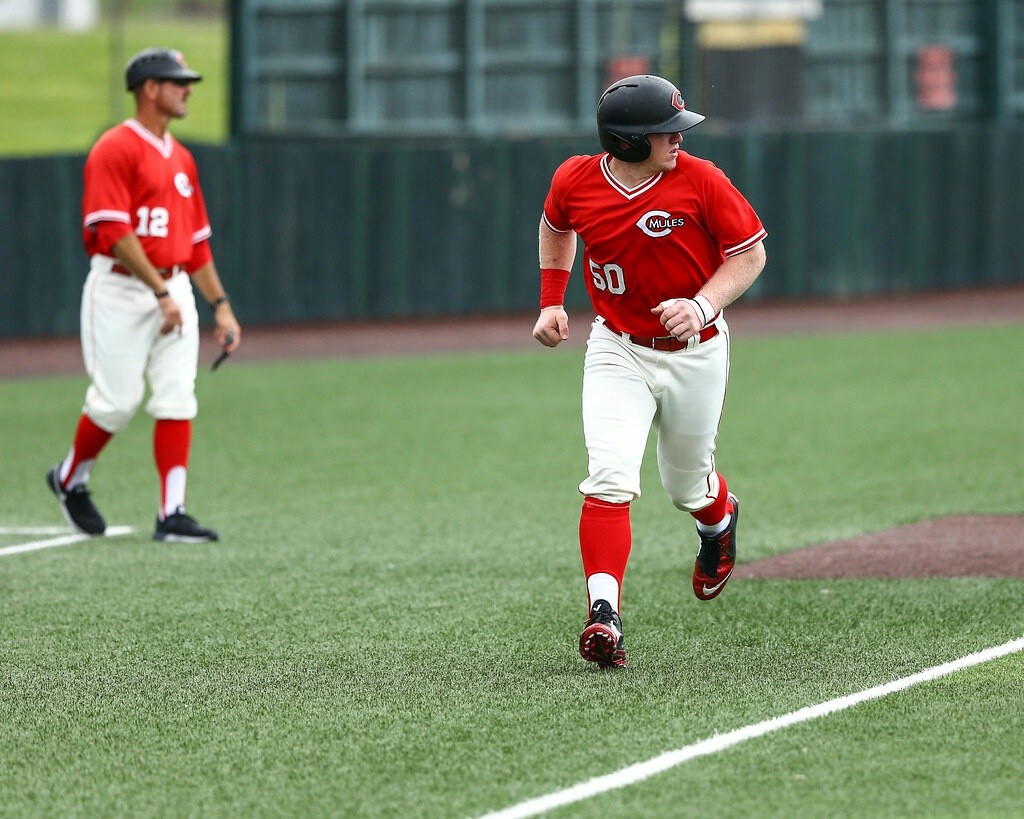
604;319;719;352
111;263;181;281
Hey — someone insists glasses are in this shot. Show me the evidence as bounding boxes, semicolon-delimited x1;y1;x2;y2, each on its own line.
175;79;190;85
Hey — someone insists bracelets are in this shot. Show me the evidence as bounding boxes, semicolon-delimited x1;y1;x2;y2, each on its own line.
211;296;230;310
687;298;706;328
154;290;169;298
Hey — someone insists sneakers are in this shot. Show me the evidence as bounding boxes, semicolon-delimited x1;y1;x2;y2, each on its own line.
692;492;739;601
580;599;627;670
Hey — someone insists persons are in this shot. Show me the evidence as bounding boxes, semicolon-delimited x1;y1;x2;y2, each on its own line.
47;49;240;542
533;75;769;670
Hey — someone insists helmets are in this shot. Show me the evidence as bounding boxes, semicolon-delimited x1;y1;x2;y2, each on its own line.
596;75;706;163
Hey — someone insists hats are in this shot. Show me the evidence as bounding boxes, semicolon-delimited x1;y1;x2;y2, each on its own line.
126;49;201;91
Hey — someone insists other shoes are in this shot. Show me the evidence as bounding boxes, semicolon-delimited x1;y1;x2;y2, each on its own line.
152;506;219;544
45;462;106;535
